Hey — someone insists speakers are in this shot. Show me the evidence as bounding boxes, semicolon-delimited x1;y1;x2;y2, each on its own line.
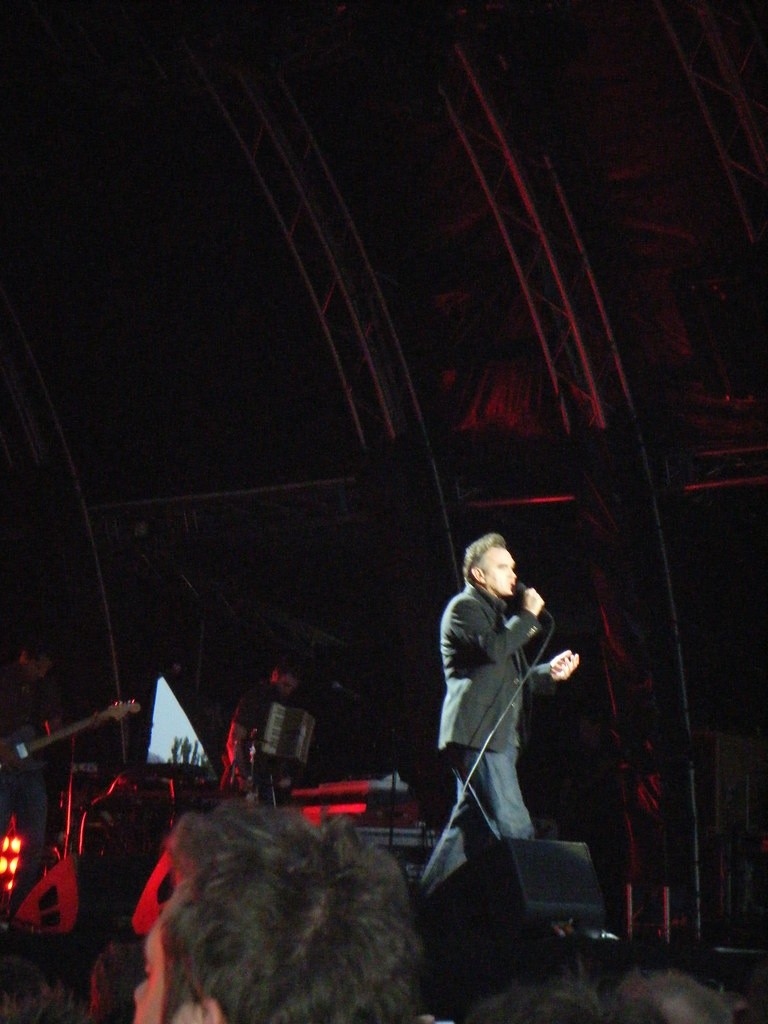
14;853;171;946
412;837;606;942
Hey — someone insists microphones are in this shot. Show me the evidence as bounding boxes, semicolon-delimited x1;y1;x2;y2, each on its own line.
513;582;551;619
332;681;360;702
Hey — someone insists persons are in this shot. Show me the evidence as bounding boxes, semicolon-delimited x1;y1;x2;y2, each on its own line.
224;640;315;802
420;532;580;896
0;810;768;1024
0;632;107;927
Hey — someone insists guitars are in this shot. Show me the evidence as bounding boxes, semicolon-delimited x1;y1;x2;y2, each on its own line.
1;696;142;766
